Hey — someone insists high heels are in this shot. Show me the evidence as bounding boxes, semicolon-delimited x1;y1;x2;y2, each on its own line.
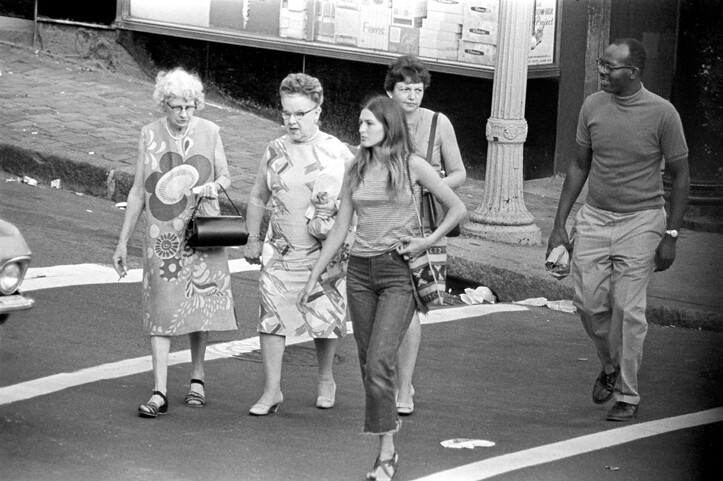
316;380;338;408
249;392;284;415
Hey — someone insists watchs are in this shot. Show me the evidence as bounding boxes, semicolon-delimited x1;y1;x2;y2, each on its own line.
664;230;679;238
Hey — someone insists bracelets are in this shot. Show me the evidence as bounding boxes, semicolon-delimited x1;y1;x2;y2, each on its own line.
249;234;261;238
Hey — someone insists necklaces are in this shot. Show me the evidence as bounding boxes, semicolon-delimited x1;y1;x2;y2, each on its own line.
165;117;192;140
294;124;319;143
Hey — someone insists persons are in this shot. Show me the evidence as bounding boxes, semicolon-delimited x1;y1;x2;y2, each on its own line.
382;57;467;415
546;37;690;421
112;67;240;417
241;72;355;414
296;95;468;481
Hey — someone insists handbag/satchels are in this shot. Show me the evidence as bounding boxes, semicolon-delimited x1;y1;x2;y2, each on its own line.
186;181;251;249
423;184;462;240
409;235;446;314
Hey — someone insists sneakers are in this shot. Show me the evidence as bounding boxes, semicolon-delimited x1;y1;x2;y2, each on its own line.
606;403;639;421
592;366;621;404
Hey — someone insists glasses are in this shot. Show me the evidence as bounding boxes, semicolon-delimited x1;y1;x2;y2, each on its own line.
166;100;197;114
596;56;637;74
280;105;318;119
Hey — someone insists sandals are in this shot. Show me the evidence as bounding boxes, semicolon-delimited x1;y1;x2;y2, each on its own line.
139;390;169;416
365;452;399;481
396;385;415;414
185;378;208;407
